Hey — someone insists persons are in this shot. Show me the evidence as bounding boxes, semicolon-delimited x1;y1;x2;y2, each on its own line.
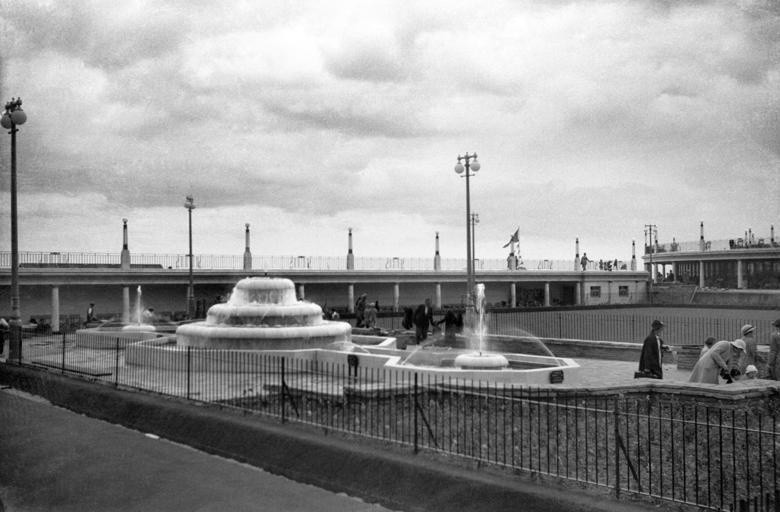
436;306;457;336
638;320;672;379
581;252;594;271
331;309;340;319
598;259;627;271
667;273;669;281
413;297;436;345
39;318;50;334
669;270;674;281
688;319;780;384
507;252;518;271
356;293;380;328
402;305;413;329
87;302;97;322
143;308;155;323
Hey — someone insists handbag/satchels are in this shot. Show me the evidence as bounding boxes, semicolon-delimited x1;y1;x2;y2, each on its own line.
634;371;657;378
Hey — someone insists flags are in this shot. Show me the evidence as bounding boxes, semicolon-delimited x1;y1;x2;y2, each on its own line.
503;229;519;248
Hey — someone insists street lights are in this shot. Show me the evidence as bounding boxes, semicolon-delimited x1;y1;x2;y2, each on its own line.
453;149;481;328
184;192;197;317
0;96;27;366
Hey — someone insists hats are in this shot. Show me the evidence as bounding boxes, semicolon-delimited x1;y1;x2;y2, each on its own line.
771;319;780;326
651;320;663;330
745;364;758;374
731;338;747;354
742;324;755;335
